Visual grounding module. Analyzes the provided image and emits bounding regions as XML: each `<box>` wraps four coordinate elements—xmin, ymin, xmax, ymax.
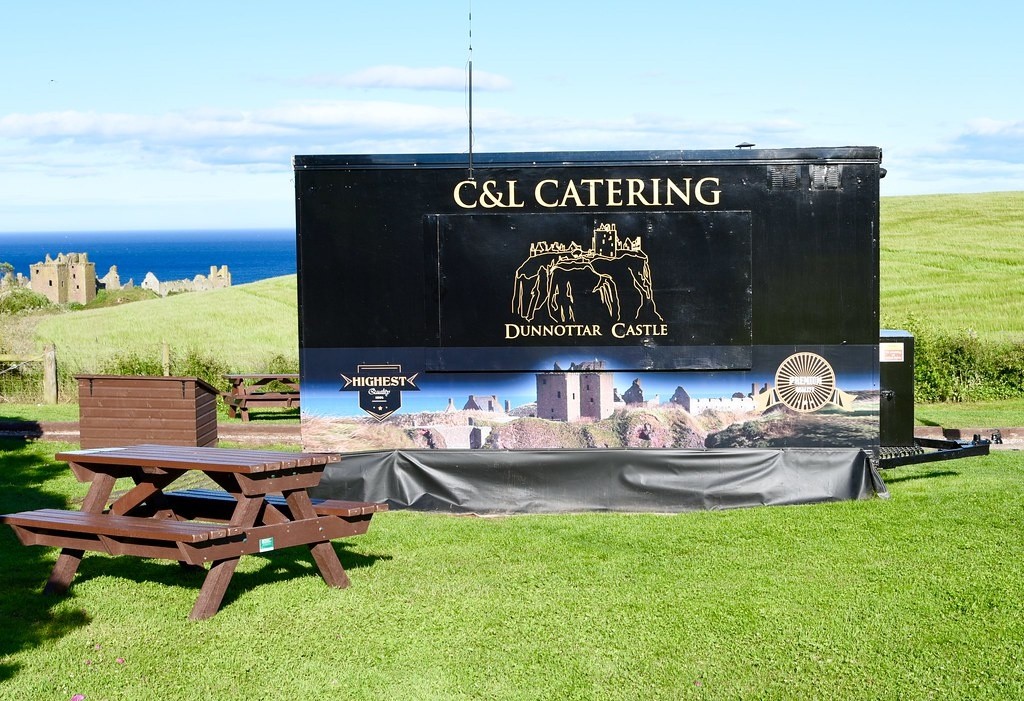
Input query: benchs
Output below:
<box><xmin>221</xmin><ymin>392</ymin><xmax>299</xmax><ymax>405</ymax></box>
<box><xmin>233</xmin><ymin>395</ymin><xmax>299</xmax><ymax>409</ymax></box>
<box><xmin>164</xmin><ymin>487</ymin><xmax>390</xmax><ymax>536</ymax></box>
<box><xmin>0</xmin><ymin>509</ymin><xmax>243</xmax><ymax>566</ymax></box>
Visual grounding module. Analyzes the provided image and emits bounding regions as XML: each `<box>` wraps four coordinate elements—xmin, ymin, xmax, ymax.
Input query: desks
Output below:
<box><xmin>222</xmin><ymin>374</ymin><xmax>299</xmax><ymax>422</ymax></box>
<box><xmin>42</xmin><ymin>445</ymin><xmax>350</xmax><ymax>622</ymax></box>
